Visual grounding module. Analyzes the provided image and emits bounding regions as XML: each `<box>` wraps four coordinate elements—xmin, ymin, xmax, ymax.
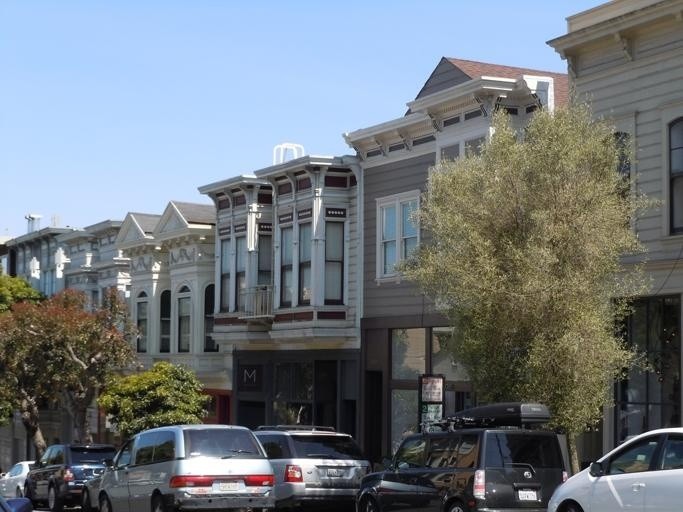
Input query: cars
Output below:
<box><xmin>0</xmin><ymin>461</ymin><xmax>35</xmax><ymax>498</ymax></box>
<box><xmin>81</xmin><ymin>476</ymin><xmax>103</xmax><ymax>512</ymax></box>
<box><xmin>620</xmin><ymin>388</ymin><xmax>652</xmax><ymax>433</ymax></box>
<box><xmin>548</xmin><ymin>427</ymin><xmax>683</xmax><ymax>512</ymax></box>
<box><xmin>0</xmin><ymin>495</ymin><xmax>33</xmax><ymax>512</ymax></box>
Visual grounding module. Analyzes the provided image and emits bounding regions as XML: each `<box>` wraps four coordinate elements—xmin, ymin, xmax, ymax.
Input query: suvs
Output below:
<box><xmin>355</xmin><ymin>402</ymin><xmax>568</xmax><ymax>512</ymax></box>
<box><xmin>253</xmin><ymin>425</ymin><xmax>373</xmax><ymax>512</ymax></box>
<box><xmin>24</xmin><ymin>444</ymin><xmax>118</xmax><ymax>512</ymax></box>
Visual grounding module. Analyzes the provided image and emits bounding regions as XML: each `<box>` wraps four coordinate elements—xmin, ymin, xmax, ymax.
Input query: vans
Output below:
<box><xmin>99</xmin><ymin>423</ymin><xmax>276</xmax><ymax>512</ymax></box>
